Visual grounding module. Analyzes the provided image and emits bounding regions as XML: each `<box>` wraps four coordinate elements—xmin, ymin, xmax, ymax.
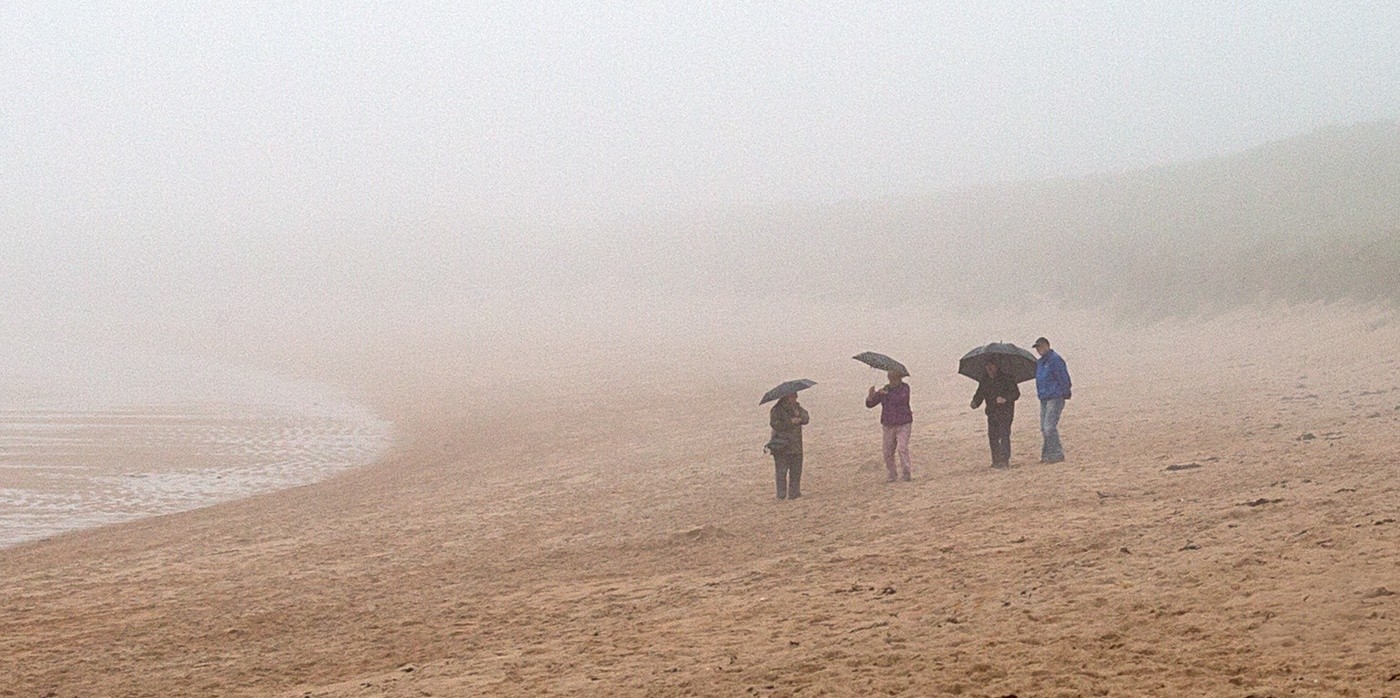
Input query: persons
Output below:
<box><xmin>865</xmin><ymin>370</ymin><xmax>914</xmax><ymax>483</ymax></box>
<box><xmin>1030</xmin><ymin>338</ymin><xmax>1073</xmax><ymax>463</ymax></box>
<box><xmin>967</xmin><ymin>355</ymin><xmax>1023</xmax><ymax>468</ymax></box>
<box><xmin>765</xmin><ymin>391</ymin><xmax>809</xmax><ymax>499</ymax></box>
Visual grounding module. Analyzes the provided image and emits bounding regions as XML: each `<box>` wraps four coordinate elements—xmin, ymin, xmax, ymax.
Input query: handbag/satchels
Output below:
<box><xmin>763</xmin><ymin>435</ymin><xmax>790</xmax><ymax>454</ymax></box>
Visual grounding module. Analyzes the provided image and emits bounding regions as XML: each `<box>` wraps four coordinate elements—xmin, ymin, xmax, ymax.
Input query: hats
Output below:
<box><xmin>1031</xmin><ymin>337</ymin><xmax>1048</xmax><ymax>348</ymax></box>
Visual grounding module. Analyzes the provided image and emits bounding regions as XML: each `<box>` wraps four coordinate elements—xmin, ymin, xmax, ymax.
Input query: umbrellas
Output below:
<box><xmin>852</xmin><ymin>351</ymin><xmax>911</xmax><ymax>391</ymax></box>
<box><xmin>758</xmin><ymin>377</ymin><xmax>818</xmax><ymax>418</ymax></box>
<box><xmin>958</xmin><ymin>339</ymin><xmax>1040</xmax><ymax>384</ymax></box>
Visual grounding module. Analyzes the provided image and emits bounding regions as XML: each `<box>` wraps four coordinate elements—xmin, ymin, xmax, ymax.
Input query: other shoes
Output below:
<box><xmin>1038</xmin><ymin>456</ymin><xmax>1064</xmax><ymax>465</ymax></box>
